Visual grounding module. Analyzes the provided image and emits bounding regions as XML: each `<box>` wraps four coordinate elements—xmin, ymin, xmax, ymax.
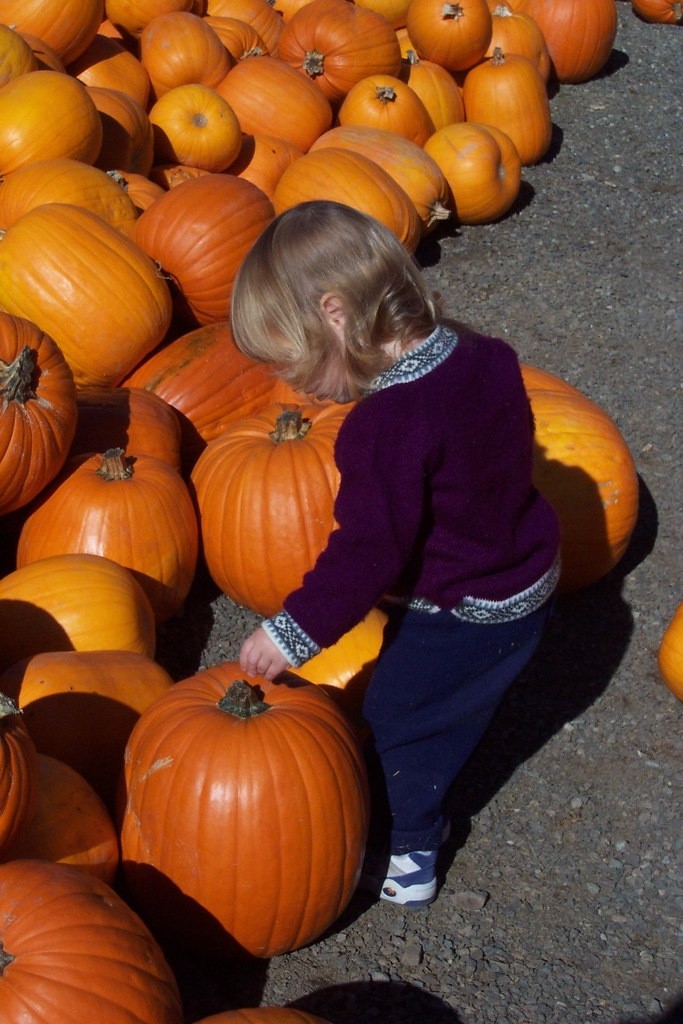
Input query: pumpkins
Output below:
<box><xmin>0</xmin><ymin>0</ymin><xmax>683</xmax><ymax>1024</ymax></box>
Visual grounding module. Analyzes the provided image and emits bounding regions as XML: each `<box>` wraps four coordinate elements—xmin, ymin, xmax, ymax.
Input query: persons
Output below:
<box><xmin>228</xmin><ymin>200</ymin><xmax>564</xmax><ymax>905</ymax></box>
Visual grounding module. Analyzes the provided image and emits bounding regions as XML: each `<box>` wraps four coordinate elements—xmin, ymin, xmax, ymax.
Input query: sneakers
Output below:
<box><xmin>360</xmin><ymin>849</ymin><xmax>441</xmax><ymax>907</ymax></box>
